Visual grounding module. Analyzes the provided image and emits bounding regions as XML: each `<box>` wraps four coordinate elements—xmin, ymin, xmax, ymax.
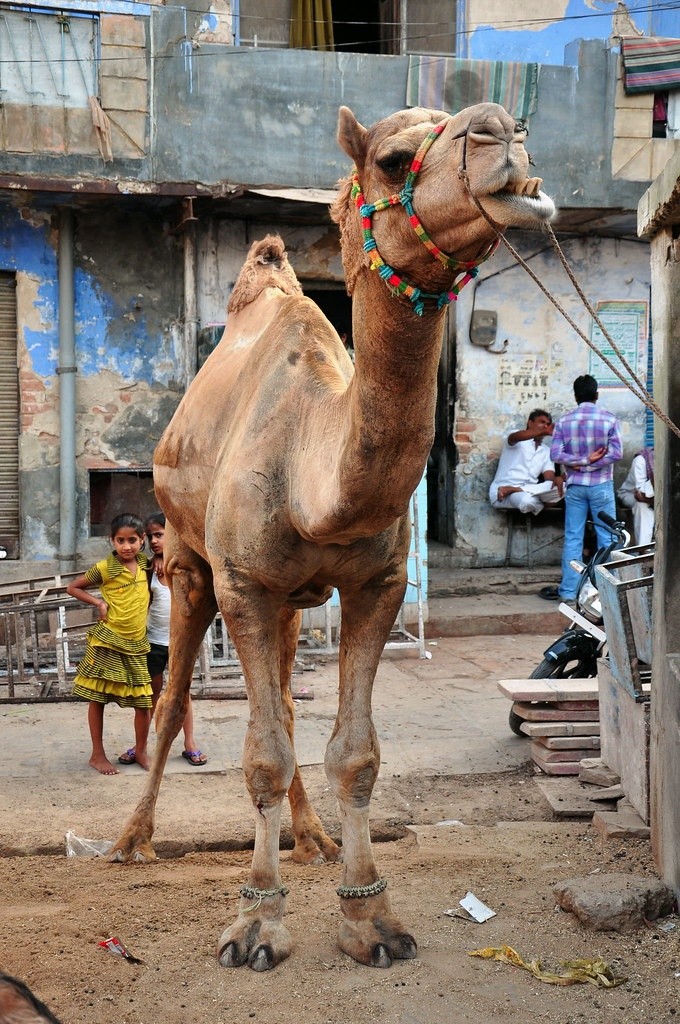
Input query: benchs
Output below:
<box><xmin>501</xmin><ymin>508</ymin><xmax>636</xmax><ymax>566</ymax></box>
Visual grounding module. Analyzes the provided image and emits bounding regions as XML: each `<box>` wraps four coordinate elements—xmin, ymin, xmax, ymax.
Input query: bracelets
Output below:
<box><xmin>96</xmin><ymin>599</ymin><xmax>103</xmax><ymax>608</ymax></box>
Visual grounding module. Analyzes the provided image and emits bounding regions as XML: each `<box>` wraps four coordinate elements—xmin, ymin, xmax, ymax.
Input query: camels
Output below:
<box><xmin>110</xmin><ymin>104</ymin><xmax>560</xmax><ymax>972</ymax></box>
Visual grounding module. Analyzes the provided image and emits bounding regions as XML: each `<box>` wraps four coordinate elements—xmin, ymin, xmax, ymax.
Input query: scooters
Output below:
<box><xmin>508</xmin><ymin>510</ymin><xmax>651</xmax><ymax>738</ymax></box>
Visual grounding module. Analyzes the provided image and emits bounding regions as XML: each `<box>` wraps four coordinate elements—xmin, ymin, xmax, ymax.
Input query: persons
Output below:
<box><xmin>488</xmin><ymin>408</ymin><xmax>567</xmax><ymax>515</ymax></box>
<box><xmin>118</xmin><ymin>512</ymin><xmax>208</xmax><ymax>765</ymax></box>
<box><xmin>340</xmin><ymin>332</ymin><xmax>355</xmax><ymax>364</ymax></box>
<box><xmin>66</xmin><ymin>513</ymin><xmax>164</xmax><ymax>775</ymax></box>
<box><xmin>616</xmin><ymin>446</ymin><xmax>656</xmax><ymax>555</ymax></box>
<box><xmin>549</xmin><ymin>374</ymin><xmax>627</xmax><ymax>606</ymax></box>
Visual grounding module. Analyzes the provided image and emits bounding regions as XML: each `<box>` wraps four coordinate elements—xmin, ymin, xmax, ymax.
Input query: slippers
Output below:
<box><xmin>539</xmin><ymin>585</ymin><xmax>560</xmax><ymax>600</ymax></box>
<box><xmin>118</xmin><ymin>748</ymin><xmax>136</xmax><ymax>764</ymax></box>
<box><xmin>181</xmin><ymin>750</ymin><xmax>208</xmax><ymax>765</ymax></box>
